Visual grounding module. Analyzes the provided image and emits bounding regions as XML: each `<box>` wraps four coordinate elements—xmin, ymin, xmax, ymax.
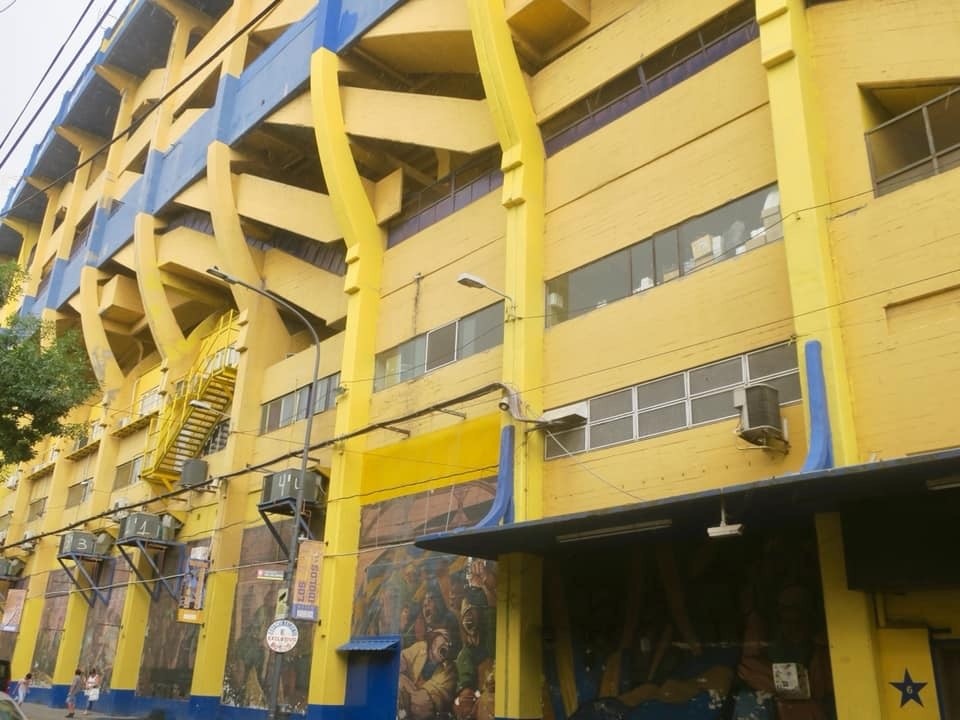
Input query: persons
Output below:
<box><xmin>82</xmin><ymin>668</ymin><xmax>101</xmax><ymax>714</ymax></box>
<box><xmin>64</xmin><ymin>670</ymin><xmax>82</xmax><ymax>719</ymax></box>
<box><xmin>11</xmin><ymin>673</ymin><xmax>33</xmax><ymax>706</ymax></box>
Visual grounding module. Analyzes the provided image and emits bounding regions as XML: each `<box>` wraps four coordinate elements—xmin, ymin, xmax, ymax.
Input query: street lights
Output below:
<box><xmin>206</xmin><ymin>266</ymin><xmax>320</xmax><ymax>717</ymax></box>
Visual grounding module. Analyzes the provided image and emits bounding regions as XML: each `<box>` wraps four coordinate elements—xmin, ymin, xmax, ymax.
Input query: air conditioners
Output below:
<box><xmin>113</xmin><ymin>497</ymin><xmax>128</xmax><ymax>522</ymax></box>
<box><xmin>7</xmin><ymin>476</ymin><xmax>17</xmax><ymax>489</ymax></box>
<box><xmin>119</xmin><ymin>417</ymin><xmax>129</xmax><ymax>426</ymax></box>
<box><xmin>191</xmin><ymin>545</ymin><xmax>210</xmax><ymax>561</ymax></box>
<box><xmin>22</xmin><ymin>531</ymin><xmax>34</xmax><ymax>550</ymax></box>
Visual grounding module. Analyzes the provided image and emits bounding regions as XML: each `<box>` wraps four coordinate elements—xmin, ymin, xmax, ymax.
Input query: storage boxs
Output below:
<box><xmin>593</xmin><ymin>190</ymin><xmax>787</xmax><ymax>308</ymax></box>
